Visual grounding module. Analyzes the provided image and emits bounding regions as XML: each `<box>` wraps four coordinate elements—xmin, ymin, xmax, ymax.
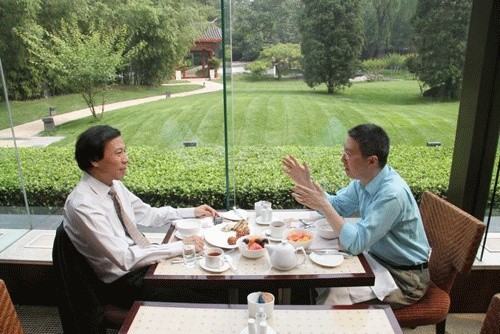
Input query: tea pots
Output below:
<box><xmin>264</xmin><ymin>240</ymin><xmax>306</xmax><ymax>271</ymax></box>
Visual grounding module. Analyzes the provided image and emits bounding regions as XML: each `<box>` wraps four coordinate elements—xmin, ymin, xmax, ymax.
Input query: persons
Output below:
<box><xmin>63</xmin><ymin>125</ymin><xmax>216</xmax><ymax>310</ymax></box>
<box><xmin>281</xmin><ymin>123</ymin><xmax>432</xmax><ymax>308</ymax></box>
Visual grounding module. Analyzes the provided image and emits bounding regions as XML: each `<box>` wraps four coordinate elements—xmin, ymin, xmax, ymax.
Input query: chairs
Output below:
<box><xmin>52</xmin><ymin>221</ymin><xmax>129</xmax><ymax>334</ymax></box>
<box><xmin>394</xmin><ymin>191</ymin><xmax>487</xmax><ymax>334</ymax></box>
<box><xmin>0</xmin><ymin>279</ymin><xmax>24</xmax><ymax>334</ymax></box>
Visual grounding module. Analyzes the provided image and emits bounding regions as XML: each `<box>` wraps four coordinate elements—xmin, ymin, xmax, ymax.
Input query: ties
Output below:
<box><xmin>108</xmin><ymin>187</ymin><xmax>150</xmax><ymax>246</ymax></box>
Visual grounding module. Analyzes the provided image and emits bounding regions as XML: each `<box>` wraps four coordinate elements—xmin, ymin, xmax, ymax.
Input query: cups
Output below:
<box><xmin>205</xmin><ymin>247</ymin><xmax>225</xmax><ymax>269</ymax></box>
<box><xmin>269</xmin><ymin>220</ymin><xmax>286</xmax><ymax>240</ymax></box>
<box><xmin>247</xmin><ymin>292</ymin><xmax>275</xmax><ymax>319</ymax></box>
<box><xmin>182</xmin><ymin>244</ymin><xmax>197</xmax><ymax>266</ymax></box>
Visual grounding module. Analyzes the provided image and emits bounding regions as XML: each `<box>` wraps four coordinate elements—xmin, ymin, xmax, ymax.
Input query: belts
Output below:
<box><xmin>391</xmin><ymin>261</ymin><xmax>430</xmax><ymax>271</ymax></box>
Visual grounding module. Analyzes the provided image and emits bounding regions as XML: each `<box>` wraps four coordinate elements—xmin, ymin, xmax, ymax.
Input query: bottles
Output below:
<box><xmin>261</xmin><ymin>204</ymin><xmax>272</xmax><ymax>222</ymax></box>
<box><xmin>248</xmin><ymin>317</ymin><xmax>267</xmax><ymax>334</ymax></box>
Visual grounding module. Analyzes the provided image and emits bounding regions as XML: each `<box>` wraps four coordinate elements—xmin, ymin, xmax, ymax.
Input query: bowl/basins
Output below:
<box><xmin>175</xmin><ymin>219</ymin><xmax>201</xmax><ymax>236</ymax></box>
<box><xmin>235</xmin><ymin>234</ymin><xmax>270</xmax><ymax>258</ymax></box>
<box><xmin>285</xmin><ymin>228</ymin><xmax>314</xmax><ymax>249</ymax></box>
<box><xmin>314</xmin><ymin>217</ymin><xmax>338</xmax><ymax>239</ymax></box>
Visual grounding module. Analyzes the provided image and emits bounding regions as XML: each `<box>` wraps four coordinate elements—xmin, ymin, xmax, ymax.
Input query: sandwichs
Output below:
<box><xmin>232</xmin><ymin>219</ymin><xmax>249</xmax><ymax>233</ymax></box>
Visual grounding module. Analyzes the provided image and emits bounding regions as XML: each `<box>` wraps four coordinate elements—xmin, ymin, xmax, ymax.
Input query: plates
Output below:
<box><xmin>204</xmin><ymin>221</ymin><xmax>255</xmax><ymax>249</ymax></box>
<box><xmin>309</xmin><ymin>251</ymin><xmax>344</xmax><ymax>267</ymax></box>
<box><xmin>256</xmin><ymin>216</ymin><xmax>276</xmax><ymax>225</ymax></box>
<box><xmin>174</xmin><ymin>229</ymin><xmax>204</xmax><ymax>240</ymax></box>
<box><xmin>239</xmin><ymin>324</ymin><xmax>277</xmax><ymax>334</ymax></box>
<box><xmin>199</xmin><ymin>257</ymin><xmax>231</xmax><ymax>272</ymax></box>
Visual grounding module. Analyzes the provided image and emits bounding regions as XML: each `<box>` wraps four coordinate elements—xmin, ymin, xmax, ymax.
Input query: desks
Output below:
<box><xmin>146</xmin><ymin>209</ymin><xmax>376</xmax><ymax>306</ymax></box>
<box><xmin>119</xmin><ymin>301</ymin><xmax>403</xmax><ymax>334</ymax></box>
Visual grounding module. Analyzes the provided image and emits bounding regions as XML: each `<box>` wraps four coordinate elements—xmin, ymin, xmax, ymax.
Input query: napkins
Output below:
<box><xmin>315</xmin><ymin>250</ymin><xmax>399</xmax><ymax>305</ymax></box>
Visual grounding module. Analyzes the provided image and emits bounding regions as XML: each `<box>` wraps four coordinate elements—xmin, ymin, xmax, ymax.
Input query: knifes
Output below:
<box><xmin>313</xmin><ymin>250</ymin><xmax>349</xmax><ymax>257</ymax></box>
